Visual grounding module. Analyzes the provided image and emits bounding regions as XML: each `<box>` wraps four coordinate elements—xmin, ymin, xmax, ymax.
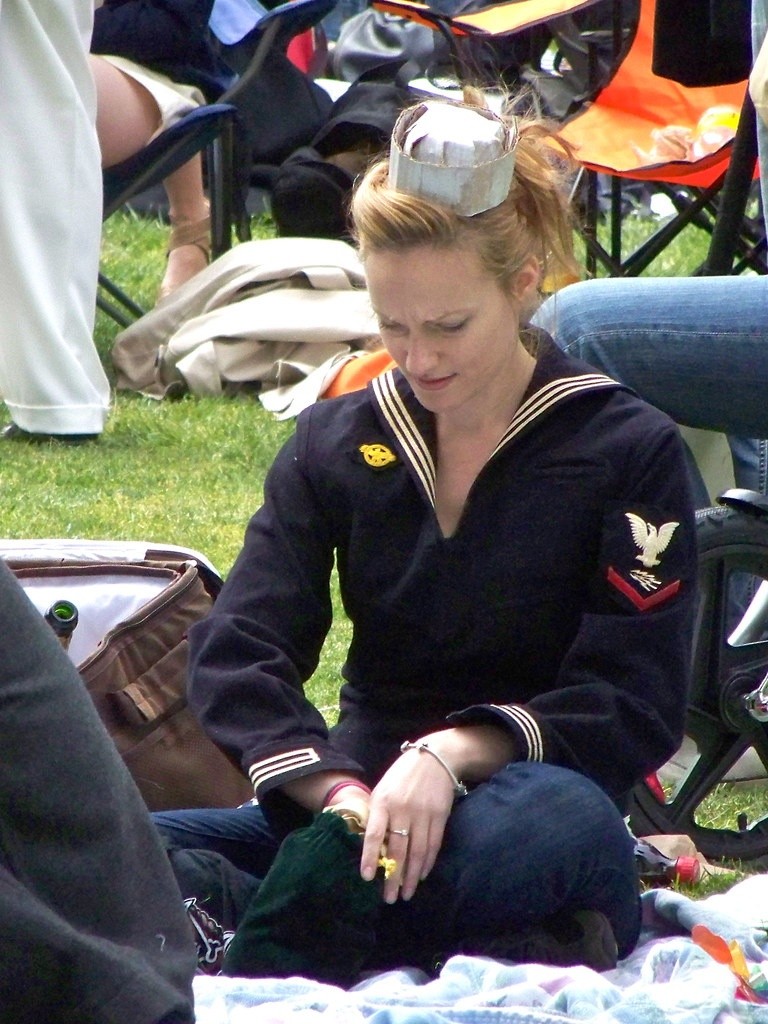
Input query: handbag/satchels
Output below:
<box><xmin>0</xmin><ymin>536</ymin><xmax>254</xmax><ymax>810</ymax></box>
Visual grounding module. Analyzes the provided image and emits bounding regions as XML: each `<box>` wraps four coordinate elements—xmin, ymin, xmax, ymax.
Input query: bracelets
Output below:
<box><xmin>323</xmin><ymin>780</ymin><xmax>372</xmax><ymax>811</ymax></box>
<box><xmin>400</xmin><ymin>740</ymin><xmax>468</xmax><ymax>796</ymax></box>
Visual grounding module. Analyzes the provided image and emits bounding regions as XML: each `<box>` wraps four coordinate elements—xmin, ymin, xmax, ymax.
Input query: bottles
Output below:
<box><xmin>45</xmin><ymin>600</ymin><xmax>78</xmax><ymax>650</ymax></box>
<box><xmin>632</xmin><ymin>836</ymin><xmax>697</xmax><ymax>889</ymax></box>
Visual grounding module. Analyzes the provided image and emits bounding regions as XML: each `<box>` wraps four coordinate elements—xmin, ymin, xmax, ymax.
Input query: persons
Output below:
<box><xmin>0</xmin><ymin>0</ymin><xmax>768</xmax><ymax>783</ymax></box>
<box><xmin>150</xmin><ymin>102</ymin><xmax>700</xmax><ymax>973</ymax></box>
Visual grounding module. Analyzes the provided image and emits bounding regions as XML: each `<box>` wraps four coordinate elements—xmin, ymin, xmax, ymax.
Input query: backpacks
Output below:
<box><xmin>279</xmin><ymin>58</ymin><xmax>420</xmax><ymax>255</ymax></box>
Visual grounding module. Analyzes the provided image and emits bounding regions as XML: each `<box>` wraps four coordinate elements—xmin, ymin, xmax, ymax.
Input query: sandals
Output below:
<box><xmin>155</xmin><ymin>210</ymin><xmax>212</xmax><ymax>308</ymax></box>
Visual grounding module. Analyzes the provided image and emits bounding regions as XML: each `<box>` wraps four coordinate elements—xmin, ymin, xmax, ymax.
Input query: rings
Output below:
<box><xmin>390</xmin><ymin>829</ymin><xmax>408</xmax><ymax>836</ymax></box>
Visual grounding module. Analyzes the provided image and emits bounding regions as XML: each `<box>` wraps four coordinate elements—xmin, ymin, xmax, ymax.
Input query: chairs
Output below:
<box><xmin>554</xmin><ymin>0</ymin><xmax>768</xmax><ymax>278</ymax></box>
<box><xmin>95</xmin><ymin>104</ymin><xmax>243</xmax><ymax>327</ymax></box>
